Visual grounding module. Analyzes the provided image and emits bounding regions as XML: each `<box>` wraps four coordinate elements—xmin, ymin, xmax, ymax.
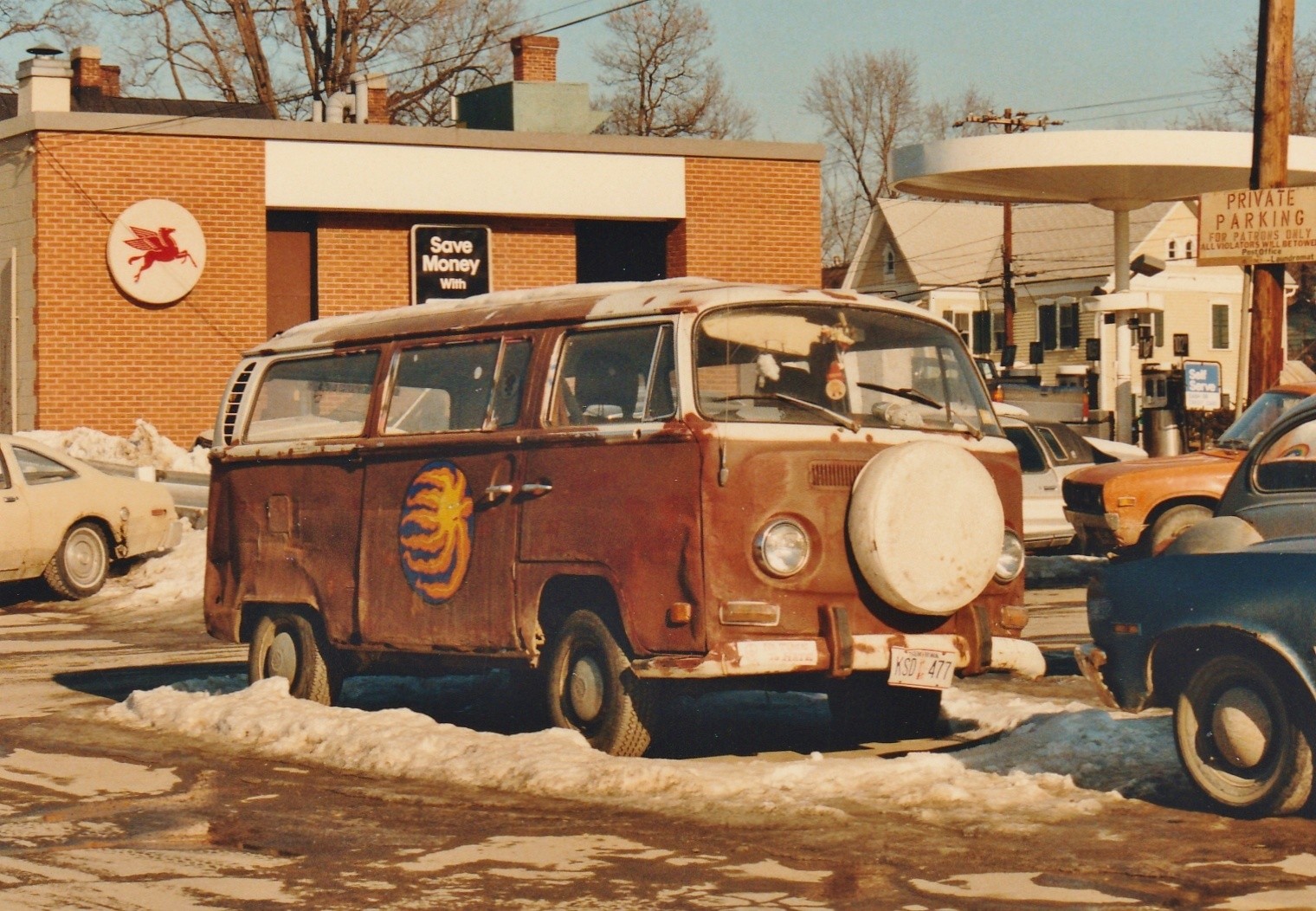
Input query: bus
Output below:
<box><xmin>201</xmin><ymin>276</ymin><xmax>1049</xmax><ymax>758</ymax></box>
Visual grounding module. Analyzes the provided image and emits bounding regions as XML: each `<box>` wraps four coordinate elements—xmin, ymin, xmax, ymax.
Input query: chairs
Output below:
<box><xmin>570</xmin><ymin>353</ymin><xmax>648</xmax><ymax>420</ymax></box>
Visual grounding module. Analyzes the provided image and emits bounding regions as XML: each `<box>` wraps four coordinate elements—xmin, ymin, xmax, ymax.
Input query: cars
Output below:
<box><xmin>1059</xmin><ymin>379</ymin><xmax>1316</xmax><ymax>558</ymax></box>
<box><xmin>1212</xmin><ymin>392</ymin><xmax>1316</xmax><ymax>540</ymax></box>
<box><xmin>0</xmin><ymin>433</ymin><xmax>183</xmax><ymax>602</ymax></box>
<box><xmin>1073</xmin><ymin>513</ymin><xmax>1315</xmax><ymax>820</ymax></box>
<box><xmin>913</xmin><ymin>397</ymin><xmax>1149</xmax><ymax>553</ymax></box>
